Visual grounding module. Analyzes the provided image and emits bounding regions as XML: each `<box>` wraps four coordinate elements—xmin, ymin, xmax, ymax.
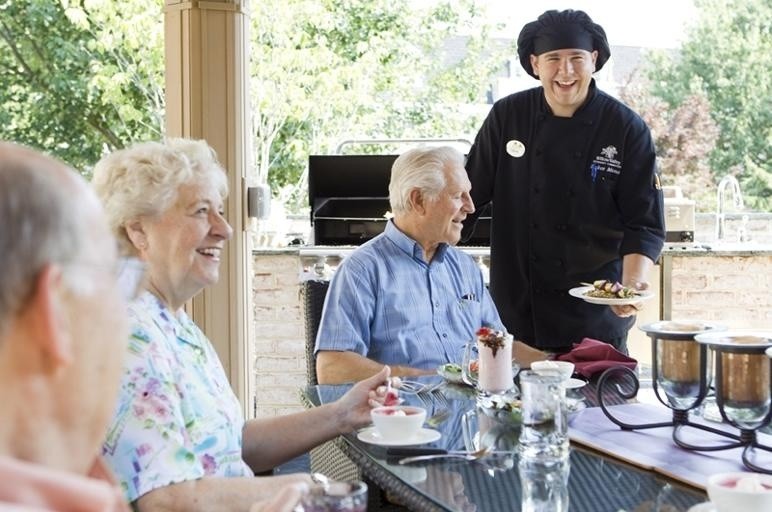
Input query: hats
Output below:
<box><xmin>517</xmin><ymin>8</ymin><xmax>611</xmax><ymax>80</ymax></box>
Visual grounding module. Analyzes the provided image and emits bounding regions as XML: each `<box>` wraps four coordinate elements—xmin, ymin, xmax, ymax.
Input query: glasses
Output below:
<box><xmin>107</xmin><ymin>255</ymin><xmax>146</xmax><ymax>299</ymax></box>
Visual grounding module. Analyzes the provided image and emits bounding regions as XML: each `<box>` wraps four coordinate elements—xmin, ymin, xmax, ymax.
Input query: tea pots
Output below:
<box><xmin>461</xmin><ymin>334</ymin><xmax>520</xmax><ymax>398</ymax></box>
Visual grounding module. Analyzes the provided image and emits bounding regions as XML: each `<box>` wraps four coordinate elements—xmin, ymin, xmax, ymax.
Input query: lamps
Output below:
<box><xmin>597</xmin><ymin>315</ymin><xmax>771</xmax><ymax>472</ymax></box>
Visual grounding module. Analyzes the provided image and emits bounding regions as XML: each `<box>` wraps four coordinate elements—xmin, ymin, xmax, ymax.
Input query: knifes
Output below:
<box><xmin>387</xmin><ymin>447</ymin><xmax>512</xmax><ymax>455</ymax></box>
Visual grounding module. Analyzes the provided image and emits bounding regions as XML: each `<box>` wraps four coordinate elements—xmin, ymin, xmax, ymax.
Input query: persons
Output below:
<box><xmin>0</xmin><ymin>139</ymin><xmax>131</xmax><ymax>510</ymax></box>
<box><xmin>313</xmin><ymin>145</ymin><xmax>552</xmax><ymax>386</ymax></box>
<box><xmin>87</xmin><ymin>137</ymin><xmax>402</xmax><ymax>511</ymax></box>
<box><xmin>458</xmin><ymin>8</ymin><xmax>665</xmax><ymax>370</ymax></box>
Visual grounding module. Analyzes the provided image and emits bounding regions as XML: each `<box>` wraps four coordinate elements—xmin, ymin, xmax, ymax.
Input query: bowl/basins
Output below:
<box><xmin>706</xmin><ymin>472</ymin><xmax>772</xmax><ymax>510</ymax></box>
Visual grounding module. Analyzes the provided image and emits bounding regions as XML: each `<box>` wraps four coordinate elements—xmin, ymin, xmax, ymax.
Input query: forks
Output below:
<box><xmin>399</xmin><ymin>449</ymin><xmax>487</xmax><ymax>465</ymax></box>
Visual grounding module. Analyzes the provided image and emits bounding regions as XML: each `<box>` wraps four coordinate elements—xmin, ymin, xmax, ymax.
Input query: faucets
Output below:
<box><xmin>717</xmin><ymin>175</ymin><xmax>743</xmax><ymax>241</ymax></box>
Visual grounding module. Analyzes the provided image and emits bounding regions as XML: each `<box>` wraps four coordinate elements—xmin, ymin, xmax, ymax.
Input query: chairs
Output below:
<box><xmin>299</xmin><ymin>277</ymin><xmax>362</xmax><ymax>482</ymax></box>
<box><xmin>716</xmin><ymin>174</ymin><xmax>768</xmax><ymax>244</ymax></box>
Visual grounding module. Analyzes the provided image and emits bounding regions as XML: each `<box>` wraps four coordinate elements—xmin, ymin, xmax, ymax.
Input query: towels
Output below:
<box><xmin>558</xmin><ymin>338</ymin><xmax>636</xmax><ymax>377</ymax></box>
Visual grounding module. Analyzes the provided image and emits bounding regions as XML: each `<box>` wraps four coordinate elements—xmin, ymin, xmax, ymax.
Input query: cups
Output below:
<box><xmin>519</xmin><ymin>371</ymin><xmax>570</xmax><ymax>461</ymax></box>
<box><xmin>461</xmin><ymin>392</ymin><xmax>520</xmax><ymax>465</ymax></box>
<box><xmin>370</xmin><ymin>405</ymin><xmax>426</xmax><ymax>439</ymax></box>
<box><xmin>531</xmin><ymin>360</ymin><xmax>575</xmax><ymax>383</ymax></box>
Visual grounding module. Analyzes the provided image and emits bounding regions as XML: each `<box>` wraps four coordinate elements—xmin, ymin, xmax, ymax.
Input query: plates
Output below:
<box><xmin>688</xmin><ymin>501</ymin><xmax>715</xmax><ymax>512</ymax></box>
<box><xmin>357</xmin><ymin>430</ymin><xmax>442</xmax><ymax>446</ymax></box>
<box><xmin>692</xmin><ymin>332</ymin><xmax>771</xmax><ymax>348</ymax></box>
<box><xmin>638</xmin><ymin>319</ymin><xmax>706</xmax><ymax>334</ymax></box>
<box><xmin>565</xmin><ymin>379</ymin><xmax>586</xmax><ymax>389</ymax></box>
<box><xmin>569</xmin><ymin>286</ymin><xmax>657</xmax><ymax>306</ymax></box>
